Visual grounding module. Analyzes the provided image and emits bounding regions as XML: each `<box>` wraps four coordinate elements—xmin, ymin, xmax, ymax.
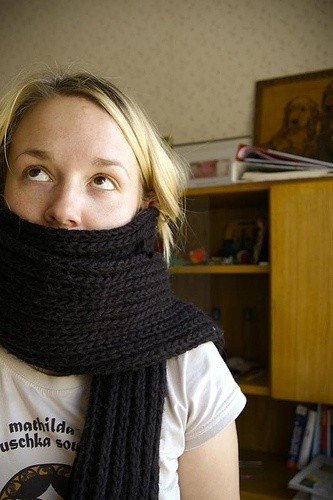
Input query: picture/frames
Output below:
<box><xmin>251</xmin><ymin>66</ymin><xmax>333</xmax><ymax>173</ymax></box>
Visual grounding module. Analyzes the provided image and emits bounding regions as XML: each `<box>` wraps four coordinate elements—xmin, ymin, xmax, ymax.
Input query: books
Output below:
<box><xmin>231</xmin><ymin>140</ymin><xmax>333</xmax><ymax>183</ymax></box>
<box><xmin>286</xmin><ymin>404</ymin><xmax>333</xmax><ymax>500</ymax></box>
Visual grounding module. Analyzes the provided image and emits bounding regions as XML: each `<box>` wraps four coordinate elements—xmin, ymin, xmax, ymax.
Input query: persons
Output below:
<box><xmin>1</xmin><ymin>73</ymin><xmax>248</xmax><ymax>499</ymax></box>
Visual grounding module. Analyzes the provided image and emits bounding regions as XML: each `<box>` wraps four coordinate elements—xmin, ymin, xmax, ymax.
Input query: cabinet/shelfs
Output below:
<box><xmin>170</xmin><ymin>174</ymin><xmax>333</xmax><ymax>500</ymax></box>
